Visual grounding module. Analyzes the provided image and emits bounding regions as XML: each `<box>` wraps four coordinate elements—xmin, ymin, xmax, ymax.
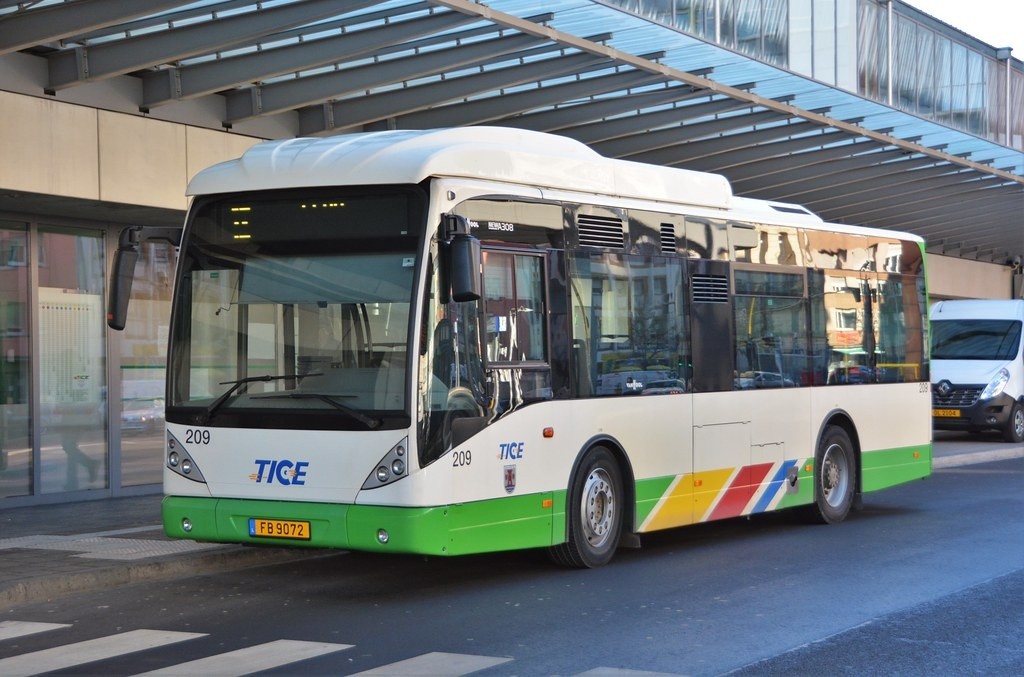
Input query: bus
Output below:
<box><xmin>107</xmin><ymin>124</ymin><xmax>935</xmax><ymax>571</ymax></box>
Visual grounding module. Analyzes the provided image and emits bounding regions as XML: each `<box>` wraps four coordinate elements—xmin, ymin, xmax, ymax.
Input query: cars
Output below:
<box><xmin>592</xmin><ymin>359</ymin><xmax>906</xmax><ymax>398</ymax></box>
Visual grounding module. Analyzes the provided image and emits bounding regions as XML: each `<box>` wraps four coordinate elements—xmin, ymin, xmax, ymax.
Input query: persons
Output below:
<box><xmin>55</xmin><ymin>357</ymin><xmax>100</xmax><ymax>493</ymax></box>
<box><xmin>433</xmin><ymin>319</ymin><xmax>486</xmax><ymax>399</ymax></box>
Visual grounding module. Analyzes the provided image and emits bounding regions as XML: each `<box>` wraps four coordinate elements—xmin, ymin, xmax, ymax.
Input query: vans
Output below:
<box><xmin>926</xmin><ymin>299</ymin><xmax>1024</xmax><ymax>445</ymax></box>
